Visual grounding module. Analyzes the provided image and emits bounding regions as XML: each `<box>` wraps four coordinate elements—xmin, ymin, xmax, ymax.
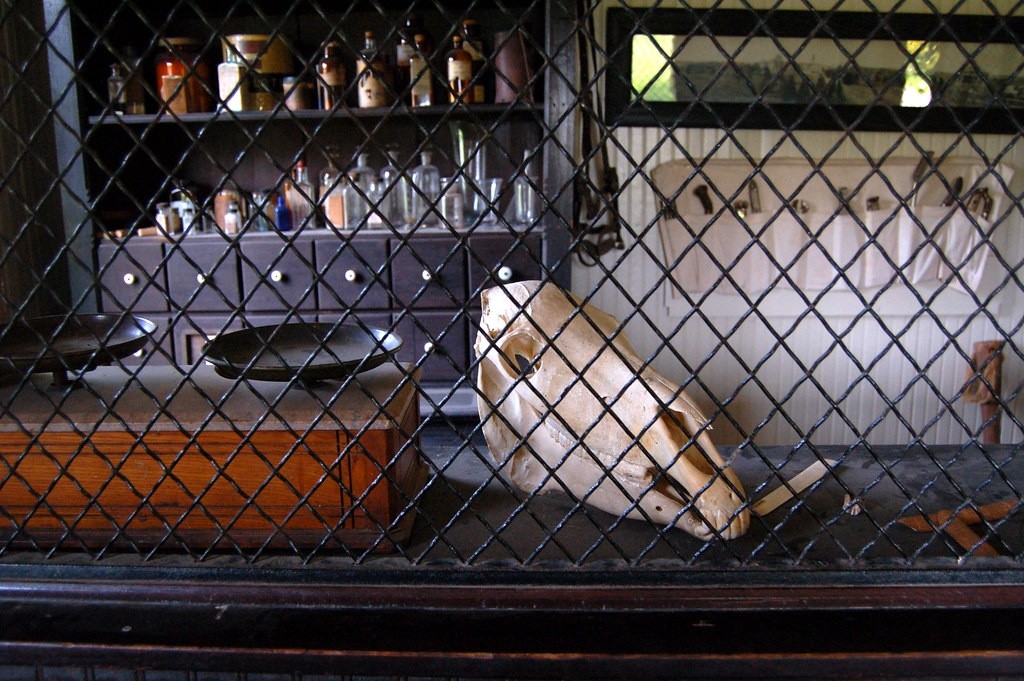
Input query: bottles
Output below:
<box><xmin>108</xmin><ymin>20</ymin><xmax>532</xmax><ymax>112</ymax></box>
<box><xmin>154</xmin><ymin>123</ymin><xmax>536</xmax><ymax>238</ymax></box>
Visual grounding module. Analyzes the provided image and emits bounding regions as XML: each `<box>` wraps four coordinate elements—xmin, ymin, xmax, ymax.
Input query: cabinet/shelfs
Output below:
<box><xmin>43</xmin><ymin>0</ymin><xmax>577</xmax><ymax>447</ymax></box>
